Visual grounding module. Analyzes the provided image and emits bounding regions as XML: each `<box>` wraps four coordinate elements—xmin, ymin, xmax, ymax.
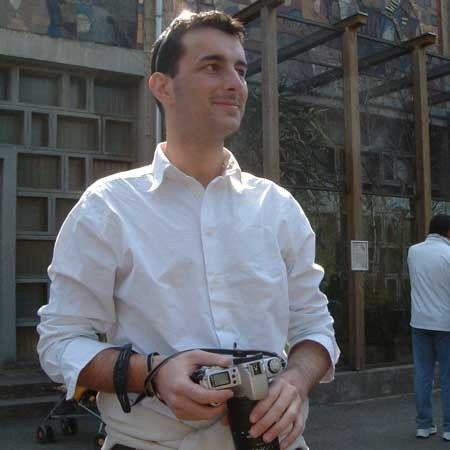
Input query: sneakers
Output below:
<box><xmin>417</xmin><ymin>425</ymin><xmax>450</xmax><ymax>440</ymax></box>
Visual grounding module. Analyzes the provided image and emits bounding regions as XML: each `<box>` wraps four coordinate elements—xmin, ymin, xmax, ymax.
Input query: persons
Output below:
<box><xmin>407</xmin><ymin>213</ymin><xmax>450</xmax><ymax>441</ymax></box>
<box><xmin>34</xmin><ymin>9</ymin><xmax>341</xmax><ymax>450</ymax></box>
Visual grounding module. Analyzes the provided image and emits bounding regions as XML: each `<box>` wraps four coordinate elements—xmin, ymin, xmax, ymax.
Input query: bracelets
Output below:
<box><xmin>148</xmin><ymin>351</ymin><xmax>159</xmax><ymax>402</ymax></box>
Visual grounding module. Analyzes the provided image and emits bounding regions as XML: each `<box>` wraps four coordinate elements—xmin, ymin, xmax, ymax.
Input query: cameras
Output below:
<box><xmin>190</xmin><ymin>353</ymin><xmax>281</xmax><ymax>450</ymax></box>
